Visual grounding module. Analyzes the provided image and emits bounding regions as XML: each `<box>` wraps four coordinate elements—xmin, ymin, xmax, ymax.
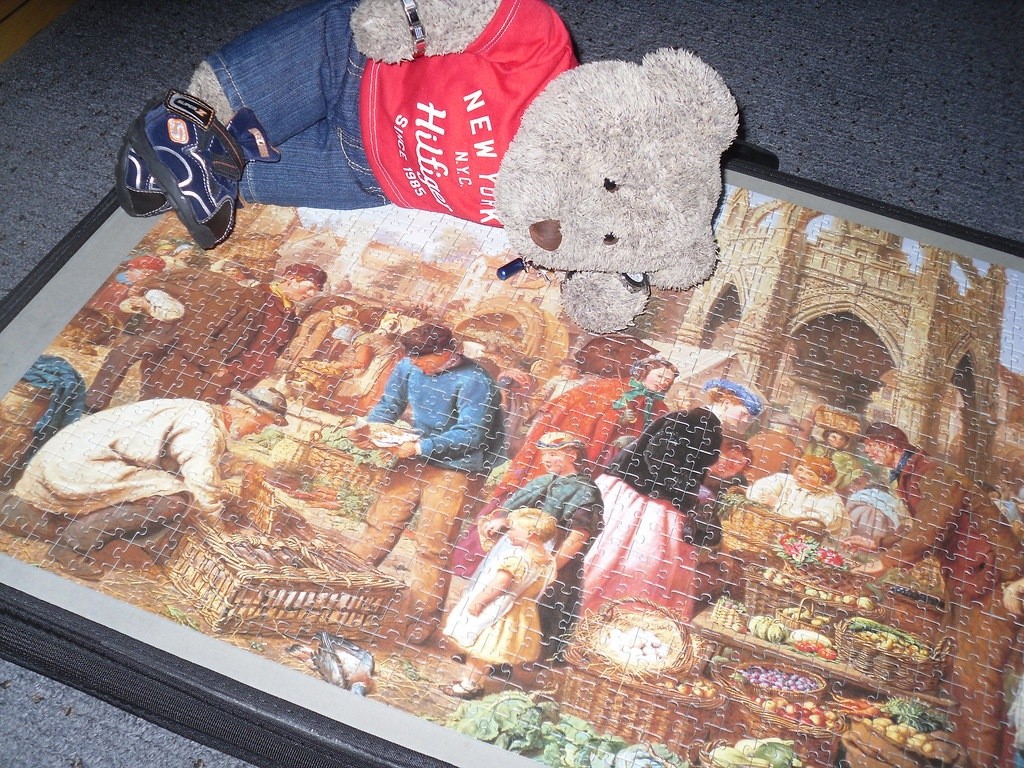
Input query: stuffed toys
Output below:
<box><xmin>114</xmin><ymin>0</ymin><xmax>739</xmax><ymax>334</ymax></box>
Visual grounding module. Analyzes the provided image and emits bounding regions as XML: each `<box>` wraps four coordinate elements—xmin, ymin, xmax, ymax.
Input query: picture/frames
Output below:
<box><xmin>0</xmin><ymin>158</ymin><xmax>1024</xmax><ymax>768</ymax></box>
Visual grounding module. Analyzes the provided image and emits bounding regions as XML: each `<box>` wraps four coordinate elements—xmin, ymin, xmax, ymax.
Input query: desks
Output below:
<box><xmin>690</xmin><ymin>605</ymin><xmax>957</xmax><ymax>708</ymax></box>
